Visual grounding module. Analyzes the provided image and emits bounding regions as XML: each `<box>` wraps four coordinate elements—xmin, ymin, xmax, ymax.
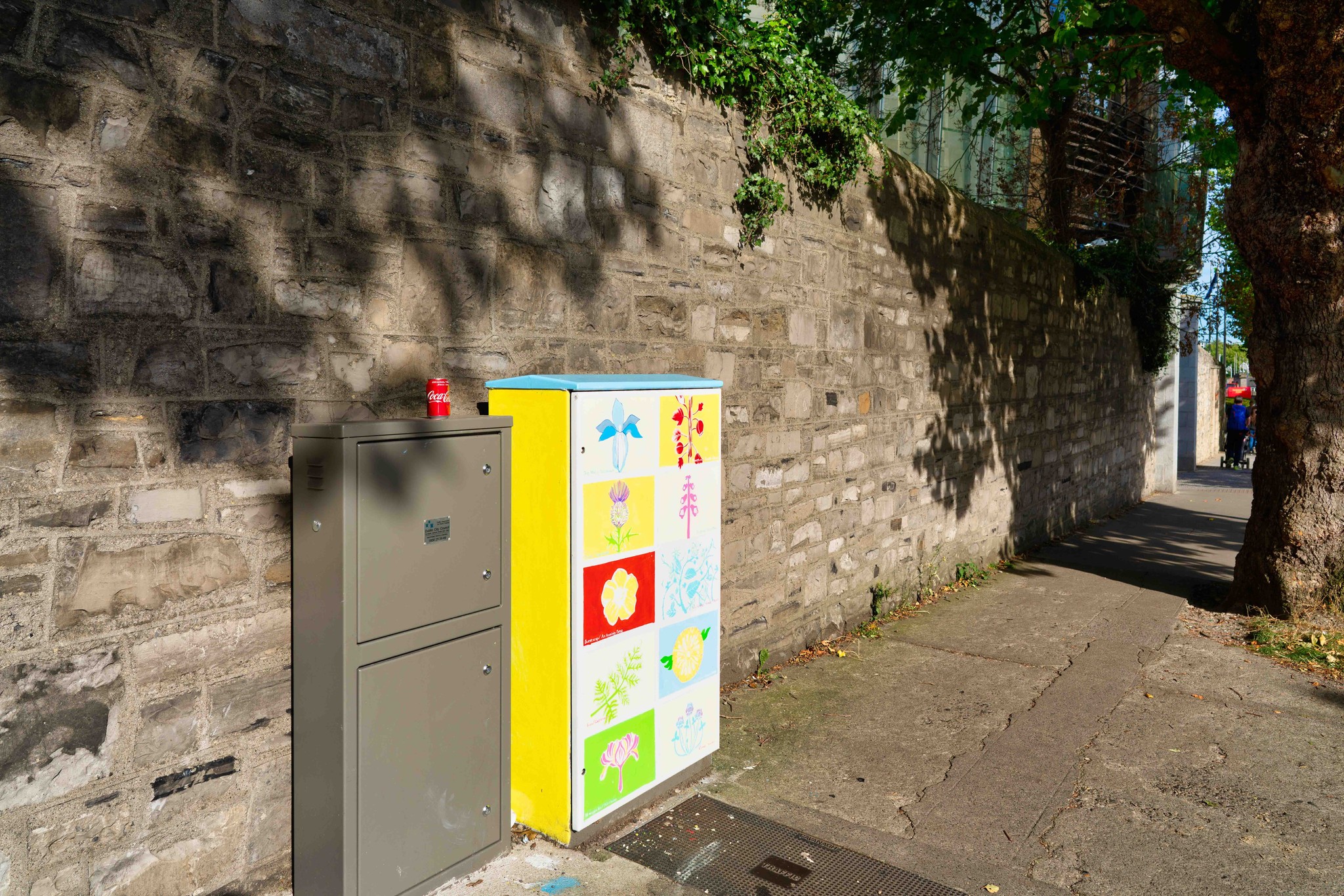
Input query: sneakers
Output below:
<box><xmin>1226</xmin><ymin>457</ymin><xmax>1234</xmax><ymax>468</ymax></box>
<box><xmin>1234</xmin><ymin>466</ymin><xmax>1243</xmax><ymax>470</ymax></box>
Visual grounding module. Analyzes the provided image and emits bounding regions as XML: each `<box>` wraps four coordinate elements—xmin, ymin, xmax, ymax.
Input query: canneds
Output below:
<box><xmin>426</xmin><ymin>378</ymin><xmax>451</xmax><ymax>418</ymax></box>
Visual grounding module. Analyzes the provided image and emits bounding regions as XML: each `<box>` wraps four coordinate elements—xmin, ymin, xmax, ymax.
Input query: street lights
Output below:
<box><xmin>1239</xmin><ymin>362</ymin><xmax>1248</xmax><ymax>387</ymax></box>
<box><xmin>1236</xmin><ymin>341</ymin><xmax>1248</xmax><ymax>364</ymax></box>
<box><xmin>1226</xmin><ymin>366</ymin><xmax>1232</xmax><ymax>378</ymax></box>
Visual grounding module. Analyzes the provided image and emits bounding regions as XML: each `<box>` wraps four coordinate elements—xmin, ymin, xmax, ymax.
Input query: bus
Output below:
<box><xmin>1226</xmin><ymin>383</ymin><xmax>1238</xmax><ymax>397</ymax></box>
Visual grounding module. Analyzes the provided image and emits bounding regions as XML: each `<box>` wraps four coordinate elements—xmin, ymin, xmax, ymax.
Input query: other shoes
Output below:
<box><xmin>1249</xmin><ymin>452</ymin><xmax>1253</xmax><ymax>455</ymax></box>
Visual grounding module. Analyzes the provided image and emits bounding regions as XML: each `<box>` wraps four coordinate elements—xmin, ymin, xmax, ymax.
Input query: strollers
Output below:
<box><xmin>1220</xmin><ymin>425</ymin><xmax>1249</xmax><ymax>469</ymax></box>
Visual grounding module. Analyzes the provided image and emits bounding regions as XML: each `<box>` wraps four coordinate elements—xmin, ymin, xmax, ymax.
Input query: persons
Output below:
<box><xmin>1225</xmin><ymin>396</ymin><xmax>1251</xmax><ymax>471</ymax></box>
<box><xmin>1246</xmin><ymin>402</ymin><xmax>1258</xmax><ymax>455</ymax></box>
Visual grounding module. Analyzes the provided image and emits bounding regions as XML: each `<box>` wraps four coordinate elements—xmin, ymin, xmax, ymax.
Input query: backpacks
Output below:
<box><xmin>1228</xmin><ymin>404</ymin><xmax>1249</xmax><ymax>436</ymax></box>
